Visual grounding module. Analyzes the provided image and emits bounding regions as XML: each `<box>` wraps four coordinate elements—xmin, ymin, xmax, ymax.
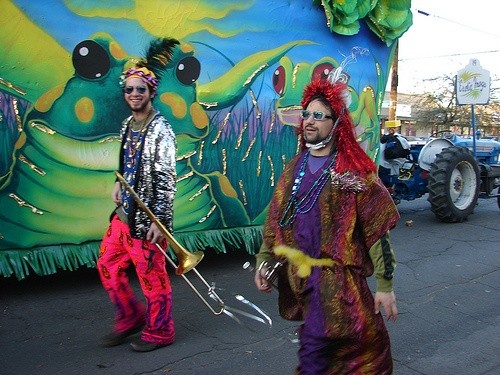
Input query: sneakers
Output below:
<box><xmin>97</xmin><ymin>323</ymin><xmax>160</xmax><ymax>352</ymax></box>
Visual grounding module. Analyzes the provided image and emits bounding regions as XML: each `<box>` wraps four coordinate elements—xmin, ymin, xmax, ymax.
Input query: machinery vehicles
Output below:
<box><xmin>377</xmin><ymin>103</ymin><xmax>500</xmax><ymax>224</ymax></box>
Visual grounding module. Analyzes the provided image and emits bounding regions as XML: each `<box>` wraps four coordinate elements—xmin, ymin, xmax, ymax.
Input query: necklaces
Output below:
<box><xmin>279</xmin><ymin>150</ymin><xmax>340</xmax><ymax>228</ymax></box>
<box><xmin>129</xmin><ymin>109</ymin><xmax>151</xmax><ymax>158</ymax></box>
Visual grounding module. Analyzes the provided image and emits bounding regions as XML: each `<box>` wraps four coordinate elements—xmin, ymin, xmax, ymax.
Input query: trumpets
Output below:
<box><xmin>257</xmin><ymin>259</ymin><xmax>283</xmax><ymax>292</ymax></box>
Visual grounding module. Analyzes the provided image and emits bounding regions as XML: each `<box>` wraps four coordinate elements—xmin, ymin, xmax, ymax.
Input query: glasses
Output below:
<box><xmin>299</xmin><ymin>110</ymin><xmax>333</xmax><ymax>121</ymax></box>
<box><xmin>122</xmin><ymin>85</ymin><xmax>147</xmax><ymax>95</ymax></box>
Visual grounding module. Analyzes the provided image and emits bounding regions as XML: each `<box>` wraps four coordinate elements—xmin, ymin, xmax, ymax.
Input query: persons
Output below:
<box><xmin>98</xmin><ymin>67</ymin><xmax>177</xmax><ymax>353</ymax></box>
<box><xmin>255</xmin><ymin>93</ymin><xmax>398</xmax><ymax>375</ymax></box>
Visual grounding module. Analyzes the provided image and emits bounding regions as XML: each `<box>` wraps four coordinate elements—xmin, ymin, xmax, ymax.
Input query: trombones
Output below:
<box><xmin>114</xmin><ymin>170</ymin><xmax>224</xmax><ymax>317</ymax></box>
